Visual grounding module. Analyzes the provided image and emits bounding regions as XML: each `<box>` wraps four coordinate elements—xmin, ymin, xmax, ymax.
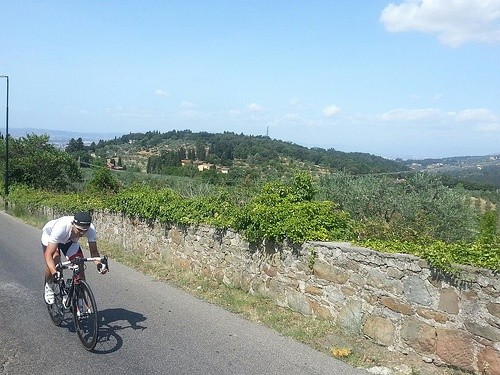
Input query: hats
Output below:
<box><xmin>74</xmin><ymin>212</ymin><xmax>91</xmax><ymax>229</ymax></box>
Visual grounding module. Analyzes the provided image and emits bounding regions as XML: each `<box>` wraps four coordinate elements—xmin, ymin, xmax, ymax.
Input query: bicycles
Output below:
<box><xmin>43</xmin><ymin>255</ymin><xmax>109</xmax><ymax>352</ymax></box>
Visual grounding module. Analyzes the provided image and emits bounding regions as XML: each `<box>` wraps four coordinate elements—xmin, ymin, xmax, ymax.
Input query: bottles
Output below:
<box><xmin>65</xmin><ymin>279</ymin><xmax>72</xmax><ymax>294</ymax></box>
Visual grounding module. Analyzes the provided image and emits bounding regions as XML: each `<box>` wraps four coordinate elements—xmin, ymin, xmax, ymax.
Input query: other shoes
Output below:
<box><xmin>76</xmin><ymin>297</ymin><xmax>86</xmax><ymax>317</ymax></box>
<box><xmin>44</xmin><ymin>282</ymin><xmax>56</xmax><ymax>305</ymax></box>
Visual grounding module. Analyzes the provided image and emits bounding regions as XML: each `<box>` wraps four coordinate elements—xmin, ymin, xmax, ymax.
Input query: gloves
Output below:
<box><xmin>52</xmin><ymin>273</ymin><xmax>64</xmax><ymax>284</ymax></box>
<box><xmin>97</xmin><ymin>263</ymin><xmax>108</xmax><ymax>274</ymax></box>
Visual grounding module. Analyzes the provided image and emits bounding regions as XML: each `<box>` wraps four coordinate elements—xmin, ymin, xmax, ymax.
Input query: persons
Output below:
<box><xmin>40</xmin><ymin>212</ymin><xmax>108</xmax><ymax>318</ymax></box>
